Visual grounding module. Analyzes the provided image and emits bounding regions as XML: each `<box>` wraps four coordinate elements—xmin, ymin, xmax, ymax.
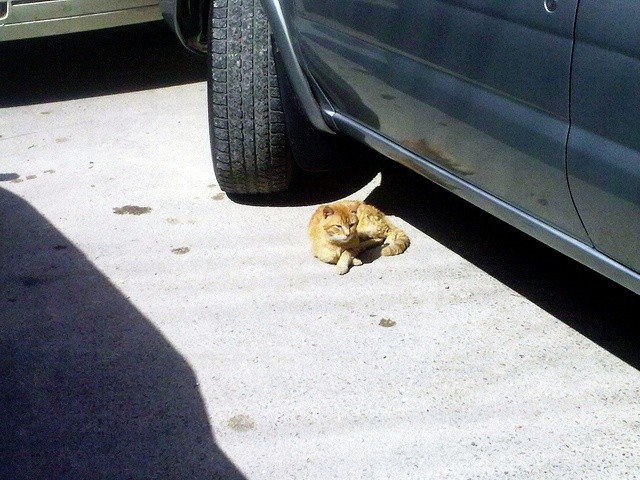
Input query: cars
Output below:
<box><xmin>0</xmin><ymin>0</ymin><xmax>212</xmax><ymax>71</ymax></box>
<box><xmin>158</xmin><ymin>0</ymin><xmax>640</xmax><ymax>294</ymax></box>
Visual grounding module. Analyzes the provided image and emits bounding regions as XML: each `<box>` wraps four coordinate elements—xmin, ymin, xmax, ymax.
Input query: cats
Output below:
<box><xmin>307</xmin><ymin>199</ymin><xmax>410</xmax><ymax>275</ymax></box>
